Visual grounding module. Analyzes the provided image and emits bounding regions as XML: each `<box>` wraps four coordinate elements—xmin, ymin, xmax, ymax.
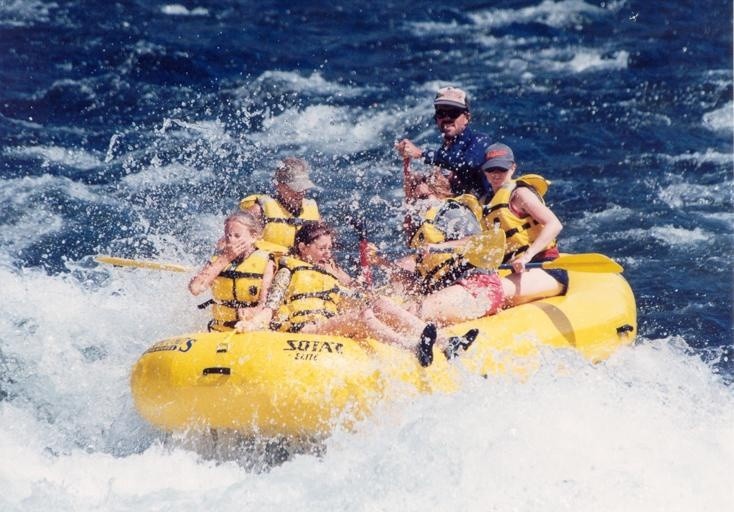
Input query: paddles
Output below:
<box><xmin>499</xmin><ymin>253</ymin><xmax>625</xmax><ymax>274</ymax></box>
<box><xmin>94</xmin><ymin>257</ymin><xmax>208</xmax><ymax>274</ymax></box>
<box><xmin>333</xmin><ymin>227</ymin><xmax>506</xmax><ymax>270</ymax></box>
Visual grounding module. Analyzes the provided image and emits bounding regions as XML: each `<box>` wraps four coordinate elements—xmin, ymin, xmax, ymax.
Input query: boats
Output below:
<box><xmin>127</xmin><ymin>250</ymin><xmax>637</xmax><ymax>435</ymax></box>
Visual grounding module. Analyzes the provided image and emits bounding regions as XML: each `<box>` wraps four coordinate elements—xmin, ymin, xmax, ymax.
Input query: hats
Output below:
<box><xmin>277</xmin><ymin>160</ymin><xmax>315</xmax><ymax>192</ymax></box>
<box><xmin>480</xmin><ymin>142</ymin><xmax>514</xmax><ymax>170</ymax></box>
<box><xmin>434</xmin><ymin>87</ymin><xmax>468</xmax><ymax>108</ymax></box>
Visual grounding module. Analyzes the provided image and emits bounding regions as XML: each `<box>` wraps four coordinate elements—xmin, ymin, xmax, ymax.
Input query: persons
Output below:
<box><xmin>391</xmin><ymin>86</ymin><xmax>494</xmax><ymax>204</ymax></box>
<box><xmin>239</xmin><ymin>158</ymin><xmax>323</xmax><ymax>262</ymax></box>
<box><xmin>187</xmin><ymin>208</ymin><xmax>278</xmax><ymax>335</ymax></box>
<box><xmin>363</xmin><ymin>168</ymin><xmax>503</xmax><ymax>328</ymax></box>
<box><xmin>235</xmin><ymin>224</ymin><xmax>481</xmax><ymax>368</ymax></box>
<box><xmin>470</xmin><ymin>139</ymin><xmax>568</xmax><ymax>310</ymax></box>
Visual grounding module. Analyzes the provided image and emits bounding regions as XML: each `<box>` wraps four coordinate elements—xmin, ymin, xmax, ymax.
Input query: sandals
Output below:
<box><xmin>416</xmin><ymin>323</ymin><xmax>436</xmax><ymax>367</ymax></box>
<box><xmin>442</xmin><ymin>328</ymin><xmax>480</xmax><ymax>362</ymax></box>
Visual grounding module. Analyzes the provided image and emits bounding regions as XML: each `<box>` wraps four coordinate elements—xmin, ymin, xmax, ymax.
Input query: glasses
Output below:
<box><xmin>436</xmin><ymin>109</ymin><xmax>460</xmax><ymax>119</ymax></box>
<box><xmin>486</xmin><ymin>166</ymin><xmax>508</xmax><ymax>172</ymax></box>
